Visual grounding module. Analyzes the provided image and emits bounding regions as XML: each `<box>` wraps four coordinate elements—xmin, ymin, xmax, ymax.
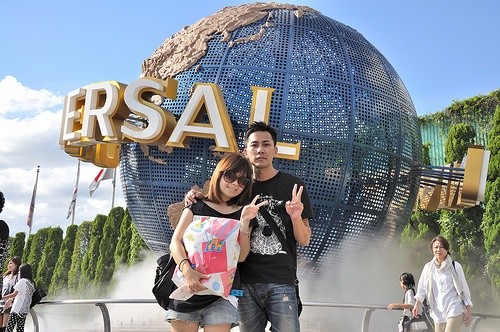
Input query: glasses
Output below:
<box><xmin>224</xmin><ymin>170</ymin><xmax>250</xmax><ymax>188</ymax></box>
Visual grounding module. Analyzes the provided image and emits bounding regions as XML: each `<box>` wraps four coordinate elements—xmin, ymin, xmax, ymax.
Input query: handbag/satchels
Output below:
<box><xmin>398</xmin><ymin>316</ymin><xmax>436</xmax><ymax>332</ymax></box>
<box><xmin>296</xmin><ymin>296</ymin><xmax>302</xmax><ymax>317</ymax></box>
<box><xmin>152</xmin><ymin>254</ymin><xmax>220</xmax><ymax>312</ymax></box>
